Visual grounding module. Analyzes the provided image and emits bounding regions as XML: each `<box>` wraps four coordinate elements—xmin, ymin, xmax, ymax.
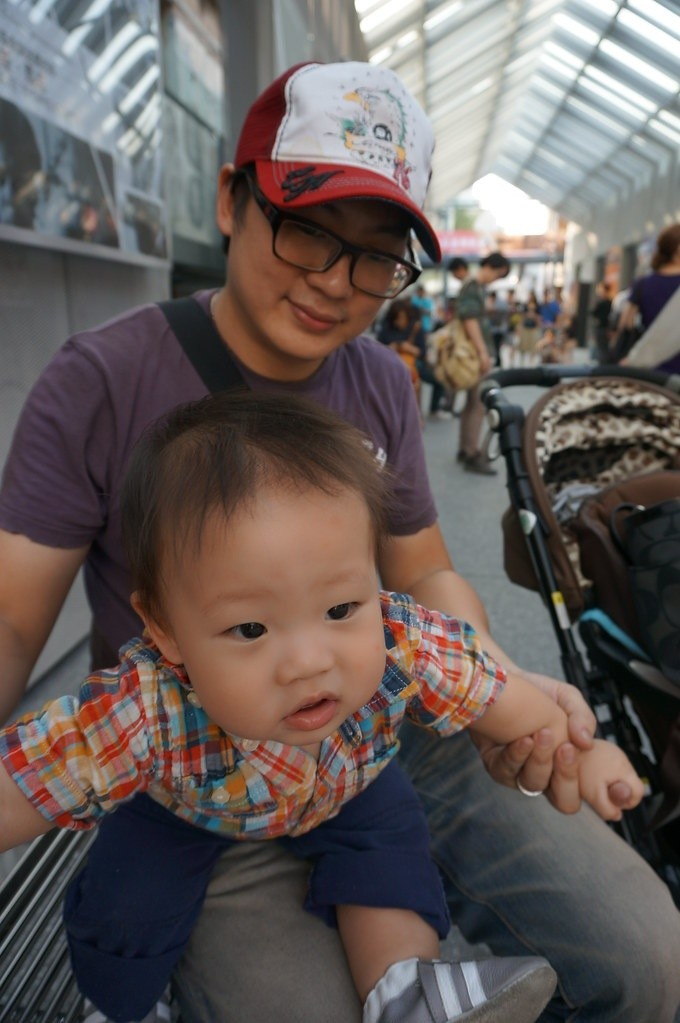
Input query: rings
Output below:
<box><xmin>515</xmin><ymin>776</ymin><xmax>544</xmax><ymax>797</ymax></box>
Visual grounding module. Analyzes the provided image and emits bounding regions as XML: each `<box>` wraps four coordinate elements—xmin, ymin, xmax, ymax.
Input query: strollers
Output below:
<box><xmin>480</xmin><ymin>361</ymin><xmax>680</xmax><ymax>902</ymax></box>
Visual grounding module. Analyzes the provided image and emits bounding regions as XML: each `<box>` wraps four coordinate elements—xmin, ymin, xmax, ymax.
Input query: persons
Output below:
<box><xmin>0</xmin><ymin>394</ymin><xmax>646</xmax><ymax>1023</ymax></box>
<box><xmin>375</xmin><ymin>220</ymin><xmax>680</xmax><ymax>476</ymax></box>
<box><xmin>0</xmin><ymin>62</ymin><xmax>680</xmax><ymax>1023</ymax></box>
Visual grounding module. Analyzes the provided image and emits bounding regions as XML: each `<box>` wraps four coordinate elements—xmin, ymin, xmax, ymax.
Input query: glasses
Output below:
<box><xmin>239</xmin><ymin>168</ymin><xmax>422</xmax><ymax>299</ymax></box>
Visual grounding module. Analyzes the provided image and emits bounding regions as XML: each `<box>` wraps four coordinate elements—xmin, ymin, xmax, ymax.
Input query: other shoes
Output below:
<box><xmin>457</xmin><ymin>449</ymin><xmax>467</xmax><ymax>463</ymax></box>
<box><xmin>378</xmin><ymin>955</ymin><xmax>558</xmax><ymax>1023</ymax></box>
<box><xmin>465</xmin><ymin>457</ymin><xmax>498</xmax><ymax>475</ymax></box>
<box><xmin>84</xmin><ymin>984</ymin><xmax>172</xmax><ymax>1023</ymax></box>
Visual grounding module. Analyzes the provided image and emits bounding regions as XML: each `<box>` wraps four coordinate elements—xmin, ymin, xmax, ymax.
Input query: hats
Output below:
<box><xmin>234</xmin><ymin>60</ymin><xmax>442</xmax><ymax>263</ymax></box>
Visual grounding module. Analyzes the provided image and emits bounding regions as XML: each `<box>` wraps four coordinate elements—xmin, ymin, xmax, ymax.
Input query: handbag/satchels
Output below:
<box><xmin>434</xmin><ymin>327</ymin><xmax>479</xmax><ymax>393</ymax></box>
<box><xmin>609</xmin><ymin>500</ymin><xmax>680</xmax><ymax>685</ymax></box>
